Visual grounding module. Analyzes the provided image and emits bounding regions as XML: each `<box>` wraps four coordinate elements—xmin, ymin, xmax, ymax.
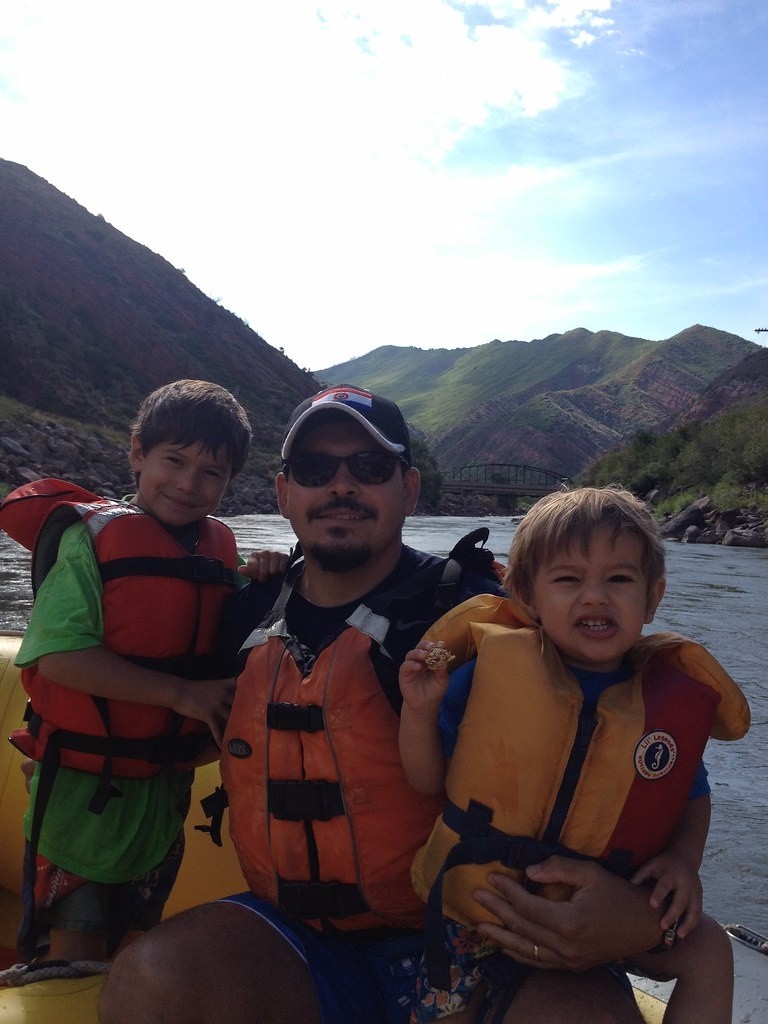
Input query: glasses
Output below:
<box><xmin>289</xmin><ymin>452</ymin><xmax>409</xmax><ymax>486</ymax></box>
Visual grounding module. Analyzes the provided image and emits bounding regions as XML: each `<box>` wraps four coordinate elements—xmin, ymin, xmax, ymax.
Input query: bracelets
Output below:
<box><xmin>646</xmin><ymin>881</ymin><xmax>680</xmax><ymax>955</ymax></box>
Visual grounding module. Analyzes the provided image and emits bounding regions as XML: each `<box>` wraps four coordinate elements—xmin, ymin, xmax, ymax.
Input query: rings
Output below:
<box><xmin>534</xmin><ymin>945</ymin><xmax>539</xmax><ymax>960</ymax></box>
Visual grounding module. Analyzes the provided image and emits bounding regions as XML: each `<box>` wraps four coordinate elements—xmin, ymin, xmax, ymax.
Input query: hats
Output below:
<box><xmin>281</xmin><ymin>383</ymin><xmax>410</xmax><ymax>466</ymax></box>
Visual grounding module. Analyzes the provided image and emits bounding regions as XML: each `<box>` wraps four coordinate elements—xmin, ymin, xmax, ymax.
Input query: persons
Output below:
<box><xmin>399</xmin><ymin>491</ymin><xmax>751</xmax><ymax>1024</ymax></box>
<box><xmin>0</xmin><ymin>379</ymin><xmax>289</xmax><ymax>963</ymax></box>
<box><xmin>20</xmin><ymin>386</ymin><xmax>680</xmax><ymax>1024</ymax></box>
